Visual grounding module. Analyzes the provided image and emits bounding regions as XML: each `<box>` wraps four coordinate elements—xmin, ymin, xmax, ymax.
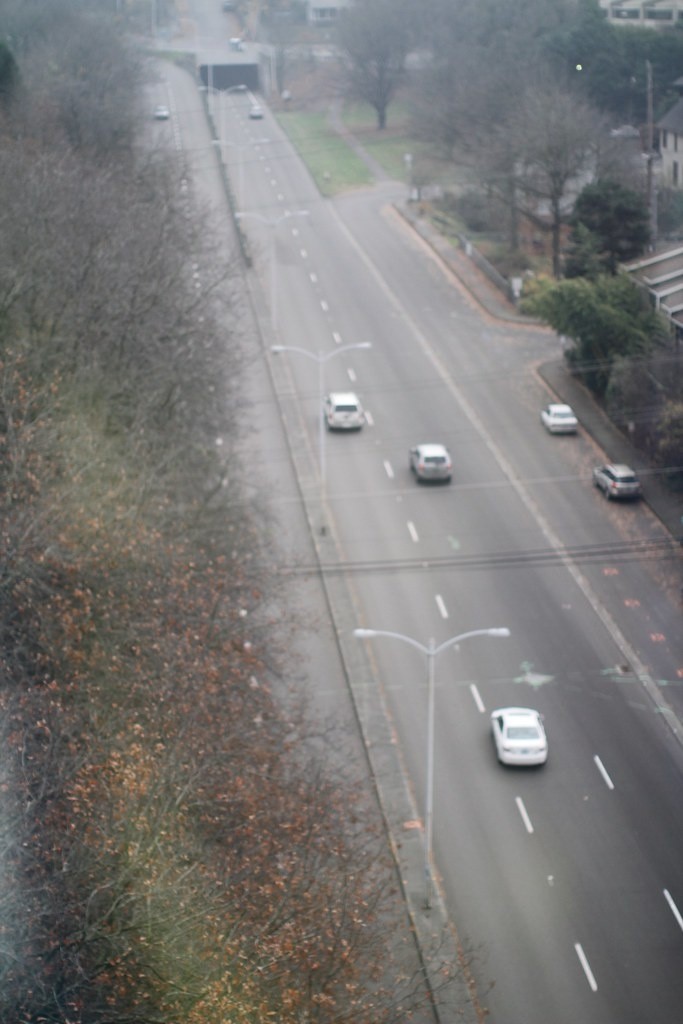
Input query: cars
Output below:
<box><xmin>154</xmin><ymin>105</ymin><xmax>169</xmax><ymax>120</ymax></box>
<box><xmin>492</xmin><ymin>707</ymin><xmax>546</xmax><ymax>767</ymax></box>
<box><xmin>328</xmin><ymin>393</ymin><xmax>363</xmax><ymax>433</ymax></box>
<box><xmin>249</xmin><ymin>106</ymin><xmax>263</xmax><ymax>119</ymax></box>
<box><xmin>541</xmin><ymin>405</ymin><xmax>576</xmax><ymax>431</ymax></box>
<box><xmin>592</xmin><ymin>463</ymin><xmax>642</xmax><ymax>498</ymax></box>
<box><xmin>411</xmin><ymin>443</ymin><xmax>451</xmax><ymax>483</ymax></box>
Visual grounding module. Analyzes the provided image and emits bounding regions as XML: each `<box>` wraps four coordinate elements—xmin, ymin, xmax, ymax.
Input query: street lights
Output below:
<box><xmin>195</xmin><ymin>83</ymin><xmax>247</xmax><ymax>134</ymax></box>
<box><xmin>272</xmin><ymin>342</ymin><xmax>372</xmax><ymax>511</ymax></box>
<box><xmin>353</xmin><ymin>627</ymin><xmax>511</xmax><ymax>863</ymax></box>
<box><xmin>210</xmin><ymin>137</ymin><xmax>264</xmax><ymax>207</ymax></box>
<box><xmin>231</xmin><ymin>210</ymin><xmax>311</xmax><ymax>331</ymax></box>
